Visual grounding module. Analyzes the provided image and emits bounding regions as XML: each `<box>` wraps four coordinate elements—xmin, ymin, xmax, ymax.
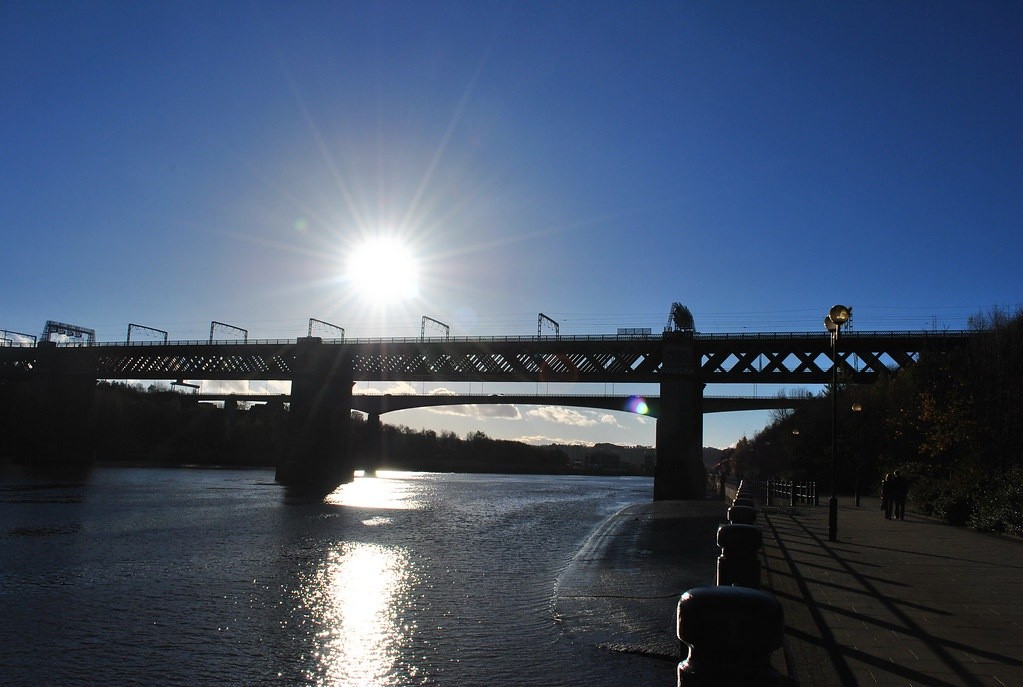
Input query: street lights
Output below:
<box><xmin>821</xmin><ymin>302</ymin><xmax>856</xmax><ymax>545</ymax></box>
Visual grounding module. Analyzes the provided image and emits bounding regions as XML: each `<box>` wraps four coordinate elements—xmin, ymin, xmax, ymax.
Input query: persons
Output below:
<box><xmin>881</xmin><ymin>471</ymin><xmax>909</xmax><ymax>520</ymax></box>
